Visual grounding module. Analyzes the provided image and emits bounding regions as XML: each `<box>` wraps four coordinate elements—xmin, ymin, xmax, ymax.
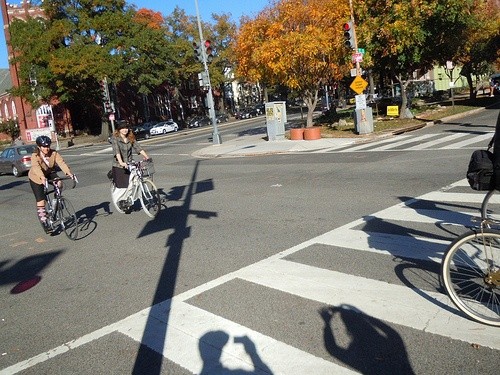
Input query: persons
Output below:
<box><xmin>111</xmin><ymin>122</ymin><xmax>150</xmax><ymax>204</ymax></box>
<box><xmin>28</xmin><ymin>136</ymin><xmax>75</xmax><ymax>228</ymax></box>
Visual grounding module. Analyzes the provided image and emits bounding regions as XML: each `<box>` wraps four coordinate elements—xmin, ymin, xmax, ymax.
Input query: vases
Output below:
<box><xmin>290</xmin><ymin>127</ymin><xmax>321</xmax><ymax>140</ymax></box>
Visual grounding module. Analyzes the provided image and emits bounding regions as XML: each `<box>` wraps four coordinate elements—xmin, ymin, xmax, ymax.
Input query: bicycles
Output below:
<box><xmin>480</xmin><ymin>187</ymin><xmax>500</xmax><ymax>245</ymax></box>
<box><xmin>37</xmin><ymin>173</ymin><xmax>79</xmax><ymax>241</ymax></box>
<box><xmin>441</xmin><ymin>216</ymin><xmax>500</xmax><ymax>328</ymax></box>
<box><xmin>110</xmin><ymin>159</ymin><xmax>161</xmax><ymax>219</ymax></box>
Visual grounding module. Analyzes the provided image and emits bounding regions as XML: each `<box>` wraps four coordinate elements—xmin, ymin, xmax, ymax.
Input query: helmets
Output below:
<box><xmin>36</xmin><ymin>136</ymin><xmax>52</xmax><ymax>146</ymax></box>
<box><xmin>117</xmin><ymin>121</ymin><xmax>129</xmax><ymax>131</ymax></box>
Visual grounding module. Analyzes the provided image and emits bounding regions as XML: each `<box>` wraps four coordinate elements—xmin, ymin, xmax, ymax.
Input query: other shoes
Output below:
<box><xmin>121</xmin><ymin>201</ymin><xmax>128</xmax><ymax>207</ymax></box>
<box><xmin>46</xmin><ymin>226</ymin><xmax>52</xmax><ymax>233</ymax></box>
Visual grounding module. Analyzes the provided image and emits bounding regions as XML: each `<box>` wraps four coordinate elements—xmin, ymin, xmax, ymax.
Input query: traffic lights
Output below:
<box><xmin>203</xmin><ymin>39</ymin><xmax>213</xmax><ymax>64</ymax></box>
<box><xmin>103</xmin><ymin>101</ymin><xmax>113</xmax><ymax>113</ymax></box>
<box><xmin>98</xmin><ymin>78</ymin><xmax>108</xmax><ymax>102</ymax></box>
<box><xmin>193</xmin><ymin>41</ymin><xmax>204</xmax><ymax>64</ymax></box>
<box><xmin>342</xmin><ymin>22</ymin><xmax>355</xmax><ymax>49</ymax></box>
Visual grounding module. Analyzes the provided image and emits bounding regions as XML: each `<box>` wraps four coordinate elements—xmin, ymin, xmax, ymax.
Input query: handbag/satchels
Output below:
<box><xmin>466</xmin><ymin>149</ymin><xmax>496</xmax><ymax>190</ymax></box>
<box><xmin>140</xmin><ymin>160</ymin><xmax>155</xmax><ymax>177</ymax></box>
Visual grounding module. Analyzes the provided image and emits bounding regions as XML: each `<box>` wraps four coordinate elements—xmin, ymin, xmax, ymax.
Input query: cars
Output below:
<box><xmin>108</xmin><ymin>125</ymin><xmax>151</xmax><ymax>144</ymax></box>
<box><xmin>0</xmin><ymin>143</ymin><xmax>38</xmax><ymax>177</ymax></box>
<box><xmin>234</xmin><ymin>103</ymin><xmax>265</xmax><ymax>120</ymax></box>
<box><xmin>150</xmin><ymin>121</ymin><xmax>179</xmax><ymax>137</ymax></box>
<box><xmin>186</xmin><ymin>113</ymin><xmax>229</xmax><ymax>129</ymax></box>
